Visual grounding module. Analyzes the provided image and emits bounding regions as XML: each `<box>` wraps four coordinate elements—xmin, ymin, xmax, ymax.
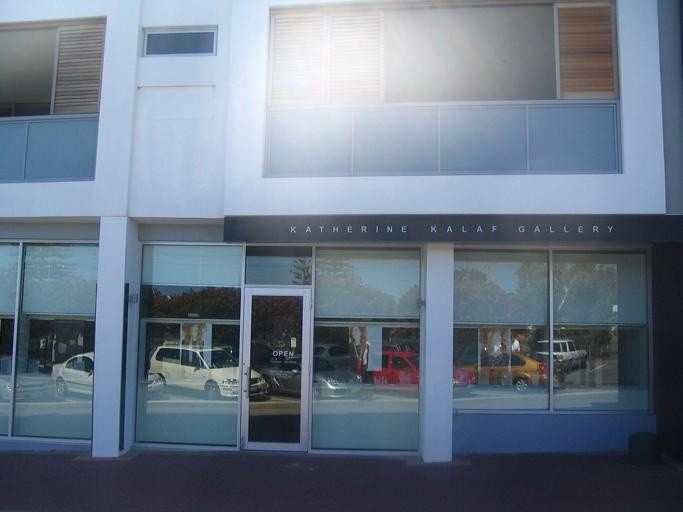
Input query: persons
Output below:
<box><xmin>350</xmin><ymin>324</ymin><xmax>369</xmax><ymax>368</ymax></box>
<box><xmin>493</xmin><ymin>338</ymin><xmax>505</xmax><ymax>352</ymax></box>
<box><xmin>512</xmin><ymin>336</ymin><xmax>521</xmax><ymax>352</ymax></box>
<box><xmin>181</xmin><ymin>320</ymin><xmax>207</xmax><ymax>367</ymax></box>
